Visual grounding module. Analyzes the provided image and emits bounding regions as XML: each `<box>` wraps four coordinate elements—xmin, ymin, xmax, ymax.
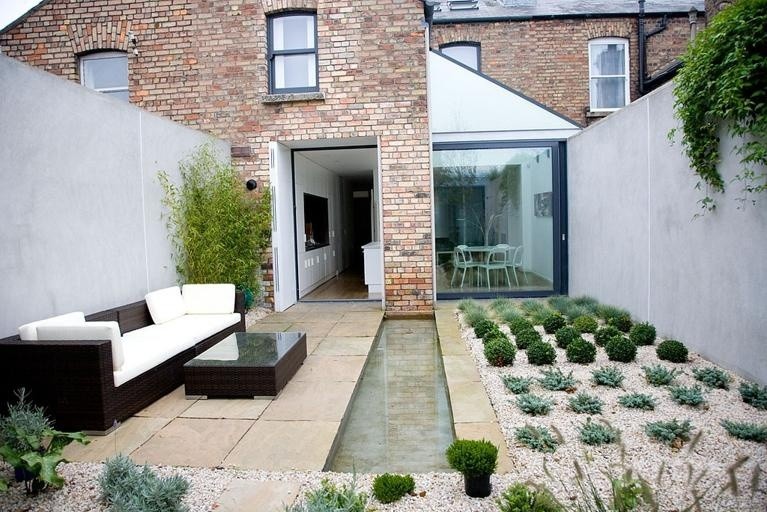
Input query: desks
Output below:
<box><xmin>181</xmin><ymin>329</ymin><xmax>308</xmax><ymax>400</ymax></box>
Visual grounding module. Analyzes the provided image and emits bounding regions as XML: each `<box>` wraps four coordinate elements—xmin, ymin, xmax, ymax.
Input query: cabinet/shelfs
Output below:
<box><xmin>292</xmin><ymin>151</ymin><xmax>350</xmax><ymax>297</ymax></box>
<box><xmin>361</xmin><ymin>241</ymin><xmax>384</xmax><ymax>295</ymax></box>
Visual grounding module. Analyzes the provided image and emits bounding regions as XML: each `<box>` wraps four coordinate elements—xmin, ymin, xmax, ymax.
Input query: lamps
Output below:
<box><xmin>246</xmin><ymin>179</ymin><xmax>257</xmax><ymax>191</ymax></box>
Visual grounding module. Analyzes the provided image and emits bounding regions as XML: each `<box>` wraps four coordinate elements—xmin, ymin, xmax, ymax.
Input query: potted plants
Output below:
<box><xmin>445</xmin><ymin>437</ymin><xmax>496</xmax><ymax>499</ymax></box>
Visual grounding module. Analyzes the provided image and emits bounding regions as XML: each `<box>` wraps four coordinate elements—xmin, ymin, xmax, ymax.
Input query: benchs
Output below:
<box><xmin>0</xmin><ymin>279</ymin><xmax>248</xmax><ymax>437</ymax></box>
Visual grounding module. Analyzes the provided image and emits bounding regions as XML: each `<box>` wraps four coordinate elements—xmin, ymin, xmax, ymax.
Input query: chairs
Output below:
<box><xmin>448</xmin><ymin>238</ymin><xmax>528</xmax><ymax>291</ymax></box>
